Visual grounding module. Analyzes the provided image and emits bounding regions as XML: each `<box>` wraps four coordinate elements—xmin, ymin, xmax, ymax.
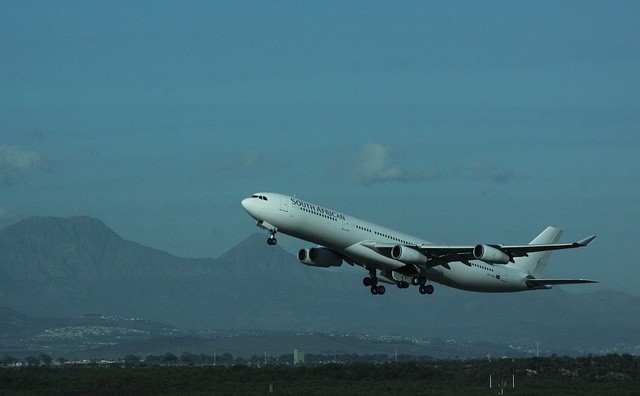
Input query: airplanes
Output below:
<box><xmin>241</xmin><ymin>191</ymin><xmax>600</xmax><ymax>294</ymax></box>
